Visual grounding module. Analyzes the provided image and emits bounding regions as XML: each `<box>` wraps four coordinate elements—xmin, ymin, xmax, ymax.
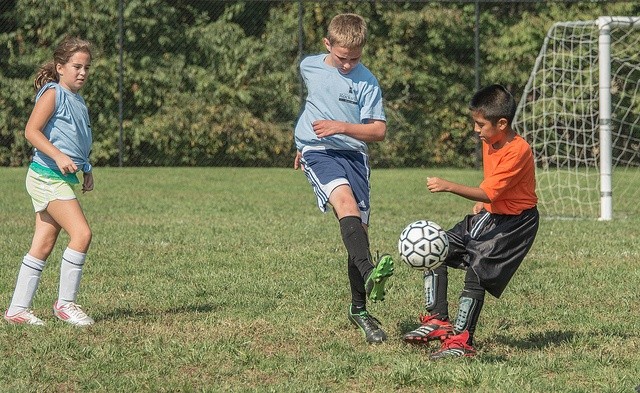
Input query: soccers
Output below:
<box><xmin>398</xmin><ymin>221</ymin><xmax>449</xmax><ymax>271</ymax></box>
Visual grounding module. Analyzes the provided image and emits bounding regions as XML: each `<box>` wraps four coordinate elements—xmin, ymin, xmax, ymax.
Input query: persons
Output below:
<box><xmin>2</xmin><ymin>34</ymin><xmax>96</xmax><ymax>325</ymax></box>
<box><xmin>293</xmin><ymin>13</ymin><xmax>395</xmax><ymax>347</ymax></box>
<box><xmin>401</xmin><ymin>84</ymin><xmax>541</xmax><ymax>361</ymax></box>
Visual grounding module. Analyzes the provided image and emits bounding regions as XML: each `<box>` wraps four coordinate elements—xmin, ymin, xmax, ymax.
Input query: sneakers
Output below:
<box><xmin>53</xmin><ymin>300</ymin><xmax>95</xmax><ymax>326</ymax></box>
<box><xmin>4</xmin><ymin>309</ymin><xmax>46</xmax><ymax>326</ymax></box>
<box><xmin>348</xmin><ymin>303</ymin><xmax>387</xmax><ymax>345</ymax></box>
<box><xmin>403</xmin><ymin>312</ymin><xmax>456</xmax><ymax>343</ymax></box>
<box><xmin>364</xmin><ymin>250</ymin><xmax>394</xmax><ymax>303</ymax></box>
<box><xmin>429</xmin><ymin>330</ymin><xmax>477</xmax><ymax>362</ymax></box>
